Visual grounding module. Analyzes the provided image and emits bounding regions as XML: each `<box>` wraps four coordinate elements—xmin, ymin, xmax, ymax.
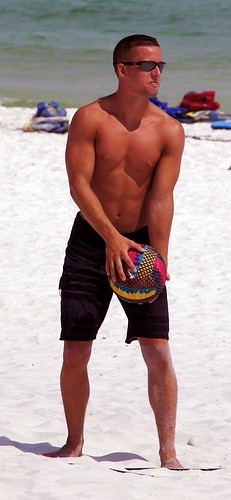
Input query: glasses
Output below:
<box><xmin>123</xmin><ymin>60</ymin><xmax>166</xmax><ymax>74</ymax></box>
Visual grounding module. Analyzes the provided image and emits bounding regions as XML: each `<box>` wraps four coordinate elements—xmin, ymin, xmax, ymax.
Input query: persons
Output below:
<box><xmin>42</xmin><ymin>34</ymin><xmax>186</xmax><ymax>470</ymax></box>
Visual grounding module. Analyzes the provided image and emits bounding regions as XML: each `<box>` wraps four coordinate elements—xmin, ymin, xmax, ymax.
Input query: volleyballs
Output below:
<box><xmin>108</xmin><ymin>244</ymin><xmax>167</xmax><ymax>303</ymax></box>
